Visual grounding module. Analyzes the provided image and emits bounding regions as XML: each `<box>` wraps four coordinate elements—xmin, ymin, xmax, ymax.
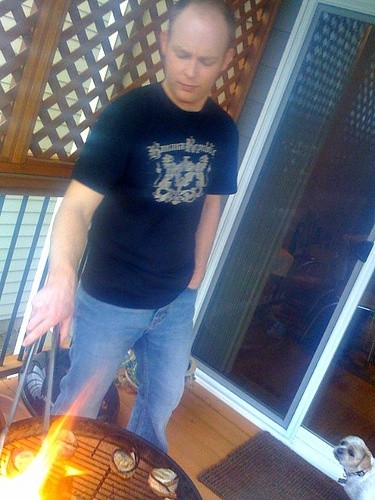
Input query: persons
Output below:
<box><xmin>19</xmin><ymin>0</ymin><xmax>242</xmax><ymax>455</ymax></box>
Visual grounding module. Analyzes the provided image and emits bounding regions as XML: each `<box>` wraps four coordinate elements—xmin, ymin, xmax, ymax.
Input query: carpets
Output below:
<box><xmin>197</xmin><ymin>431</ymin><xmax>350</xmax><ymax>500</ymax></box>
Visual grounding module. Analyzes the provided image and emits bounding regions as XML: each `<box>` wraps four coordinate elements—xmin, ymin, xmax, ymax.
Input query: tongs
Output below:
<box><xmin>0</xmin><ymin>322</ymin><xmax>60</xmax><ymax>459</ymax></box>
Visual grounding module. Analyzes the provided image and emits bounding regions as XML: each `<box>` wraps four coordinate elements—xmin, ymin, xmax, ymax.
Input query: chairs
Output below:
<box><xmin>299</xmin><ymin>286</ymin><xmax>375</xmax><ymax>368</ymax></box>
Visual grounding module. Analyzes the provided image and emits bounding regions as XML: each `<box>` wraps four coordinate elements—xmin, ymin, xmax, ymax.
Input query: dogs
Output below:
<box><xmin>333</xmin><ymin>435</ymin><xmax>375</xmax><ymax>500</ymax></box>
